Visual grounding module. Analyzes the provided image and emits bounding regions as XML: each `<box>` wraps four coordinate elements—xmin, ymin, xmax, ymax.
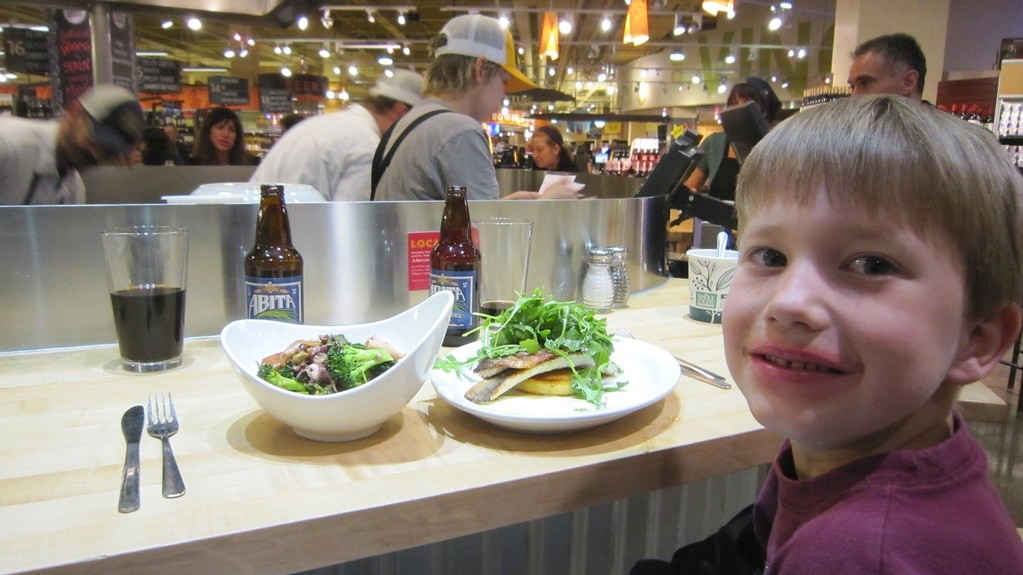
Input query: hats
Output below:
<box><xmin>745</xmin><ymin>76</ymin><xmax>782</xmax><ymax>117</ymax></box>
<box><xmin>434</xmin><ymin>14</ymin><xmax>539</xmax><ymax>93</ymax></box>
<box><xmin>79</xmin><ymin>84</ymin><xmax>144</xmax><ymax>156</ymax></box>
<box><xmin>539</xmin><ymin>126</ymin><xmax>563</xmax><ymax>147</ymax></box>
<box><xmin>369</xmin><ymin>70</ymin><xmax>425</xmax><ymax>107</ymax></box>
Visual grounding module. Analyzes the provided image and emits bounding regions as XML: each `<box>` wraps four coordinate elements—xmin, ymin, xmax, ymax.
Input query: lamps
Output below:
<box><xmin>669</xmin><ymin>17</ymin><xmax>737</xmax><ymax>94</ymax></box>
<box><xmin>538</xmin><ymin>0</ymin><xmax>560</xmax><ymax>62</ymax></box>
<box><xmin>701</xmin><ymin>0</ymin><xmax>736</xmax><ymax>19</ymax></box>
<box><xmin>318</xmin><ymin>6</ymin><xmax>417</xmax><ymax>29</ymax></box>
<box><xmin>622</xmin><ymin>0</ymin><xmax>650</xmax><ymax>47</ymax></box>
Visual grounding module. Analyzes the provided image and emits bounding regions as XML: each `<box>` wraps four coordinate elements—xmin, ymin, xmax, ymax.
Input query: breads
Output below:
<box><xmin>514</xmin><ymin>376</ymin><xmax>578</xmax><ymax>395</ymax></box>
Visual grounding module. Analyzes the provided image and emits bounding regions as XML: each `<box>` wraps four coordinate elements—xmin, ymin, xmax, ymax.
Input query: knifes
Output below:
<box><xmin>118</xmin><ymin>406</ymin><xmax>145</xmax><ymax>513</ymax></box>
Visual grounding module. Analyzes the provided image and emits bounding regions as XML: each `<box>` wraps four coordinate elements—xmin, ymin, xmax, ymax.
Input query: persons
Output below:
<box><xmin>0</xmin><ymin>82</ymin><xmax>190</xmax><ymax>205</ymax></box>
<box><xmin>531</xmin><ymin>126</ymin><xmax>581</xmax><ymax>172</ymax></box>
<box><xmin>628</xmin><ymin>92</ymin><xmax>1023</xmax><ymax>575</ymax></box>
<box><xmin>279</xmin><ymin>113</ymin><xmax>305</xmax><ymax>134</ymax></box>
<box><xmin>191</xmin><ymin>107</ymin><xmax>260</xmax><ymax>166</ymax></box>
<box><xmin>248</xmin><ymin>68</ymin><xmax>426</xmax><ymax>202</ymax></box>
<box><xmin>369</xmin><ymin>14</ymin><xmax>541</xmax><ymax>201</ymax></box>
<box><xmin>847</xmin><ymin>32</ymin><xmax>926</xmax><ymax>101</ymax></box>
<box><xmin>683</xmin><ymin>76</ymin><xmax>782</xmax><ymax>203</ymax></box>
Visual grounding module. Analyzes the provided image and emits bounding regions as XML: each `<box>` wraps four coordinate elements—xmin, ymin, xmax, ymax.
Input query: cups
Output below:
<box><xmin>469</xmin><ymin>215</ymin><xmax>540</xmax><ymax>321</ymax></box>
<box><xmin>685</xmin><ymin>248</ymin><xmax>740</xmax><ymax>323</ymax></box>
<box><xmin>100</xmin><ymin>225</ymin><xmax>192</xmax><ymax>375</ymax></box>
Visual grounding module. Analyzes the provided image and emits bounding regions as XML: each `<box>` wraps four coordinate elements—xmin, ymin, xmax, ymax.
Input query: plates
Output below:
<box><xmin>431</xmin><ymin>333</ymin><xmax>682</xmax><ymax>436</ymax></box>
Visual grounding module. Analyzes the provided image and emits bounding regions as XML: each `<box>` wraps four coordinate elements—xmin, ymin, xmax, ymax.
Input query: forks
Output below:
<box><xmin>146</xmin><ymin>391</ymin><xmax>186</xmax><ymax>499</ymax></box>
<box><xmin>609</xmin><ymin>326</ymin><xmax>728</xmax><ymax>381</ymax></box>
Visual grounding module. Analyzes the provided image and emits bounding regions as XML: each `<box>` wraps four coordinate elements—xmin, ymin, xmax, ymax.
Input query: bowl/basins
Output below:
<box><xmin>219</xmin><ymin>290</ymin><xmax>455</xmax><ymax>443</ymax></box>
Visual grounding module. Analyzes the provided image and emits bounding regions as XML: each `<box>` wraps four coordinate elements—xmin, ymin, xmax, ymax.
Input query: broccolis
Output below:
<box><xmin>256</xmin><ymin>333</ymin><xmax>399</xmax><ymax>396</ymax></box>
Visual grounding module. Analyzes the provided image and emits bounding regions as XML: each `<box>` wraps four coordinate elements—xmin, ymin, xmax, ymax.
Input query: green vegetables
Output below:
<box><xmin>433</xmin><ymin>288</ymin><xmax>630</xmax><ymax>409</ymax></box>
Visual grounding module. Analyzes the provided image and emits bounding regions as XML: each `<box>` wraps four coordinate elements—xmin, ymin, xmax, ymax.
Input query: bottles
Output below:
<box><xmin>800</xmin><ymin>85</ymin><xmax>852</xmax><ymax>113</ymax></box>
<box><xmin>550</xmin><ymin>238</ymin><xmax>599</xmax><ymax>303</ymax></box>
<box><xmin>428</xmin><ymin>185</ymin><xmax>483</xmax><ymax>348</ymax></box>
<box><xmin>580</xmin><ymin>245</ymin><xmax>633</xmax><ymax>314</ymax></box>
<box><xmin>934</xmin><ymin>103</ymin><xmax>993</xmax><ymax>133</ymax></box>
<box><xmin>24</xmin><ymin>95</ymin><xmax>52</xmax><ymax>119</ymax></box>
<box><xmin>243</xmin><ymin>185</ymin><xmax>303</xmax><ymax>326</ymax></box>
<box><xmin>631</xmin><ymin>146</ymin><xmax>663</xmax><ymax>178</ymax></box>
